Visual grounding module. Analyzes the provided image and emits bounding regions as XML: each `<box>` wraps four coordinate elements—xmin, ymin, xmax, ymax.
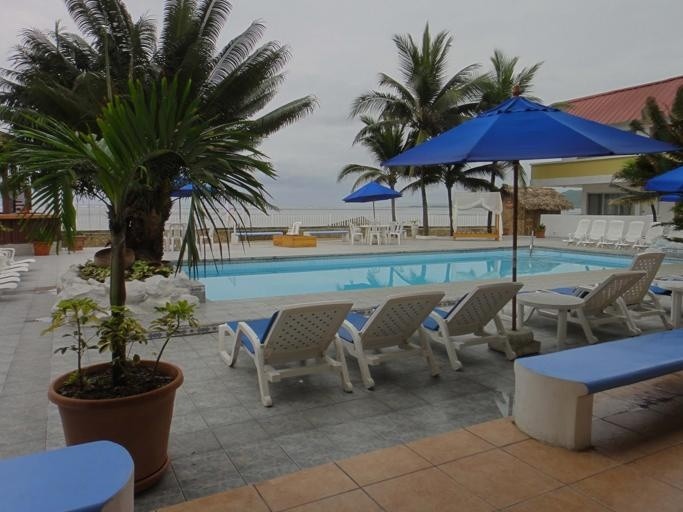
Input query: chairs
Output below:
<box><xmin>1</xmin><ymin>248</ymin><xmax>36</xmax><ymax>293</ymax></box>
<box><xmin>0</xmin><ymin>439</ymin><xmax>135</xmax><ymax>512</ymax></box>
<box><xmin>562</xmin><ymin>219</ymin><xmax>683</xmax><ymax>256</ymax></box>
<box><xmin>163</xmin><ymin>220</ymin><xmax>215</xmax><ymax>253</ymax></box>
<box><xmin>347</xmin><ymin>220</ymin><xmax>406</xmax><ymax>246</ymax></box>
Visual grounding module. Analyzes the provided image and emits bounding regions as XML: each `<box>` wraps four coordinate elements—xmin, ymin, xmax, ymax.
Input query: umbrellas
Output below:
<box><xmin>165</xmin><ymin>171</ymin><xmax>213</xmax><ymax>222</ymax></box>
<box><xmin>337</xmin><ymin>180</ymin><xmax>403</xmax><ymax>218</ymax></box>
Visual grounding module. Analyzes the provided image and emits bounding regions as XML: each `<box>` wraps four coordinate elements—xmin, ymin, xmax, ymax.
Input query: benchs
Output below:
<box><xmin>230</xmin><ymin>232</ymin><xmax>283</xmax><ymax>244</ymax></box>
<box><xmin>303</xmin><ymin>231</ymin><xmax>349</xmax><ymax>242</ymax></box>
<box><xmin>512</xmin><ymin>328</ymin><xmax>683</xmax><ymax>451</ymax></box>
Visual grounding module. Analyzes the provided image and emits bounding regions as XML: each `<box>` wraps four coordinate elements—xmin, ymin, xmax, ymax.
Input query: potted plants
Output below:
<box><xmin>534</xmin><ymin>222</ymin><xmax>546</xmax><ymax>238</ymax></box>
<box><xmin>1</xmin><ymin>71</ymin><xmax>280</xmax><ymax>496</ymax></box>
<box><xmin>26</xmin><ymin>225</ymin><xmax>57</xmax><ymax>256</ymax></box>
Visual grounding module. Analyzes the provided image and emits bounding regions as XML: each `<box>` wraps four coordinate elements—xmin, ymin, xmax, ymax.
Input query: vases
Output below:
<box><xmin>71</xmin><ymin>235</ymin><xmax>89</xmax><ymax>250</ymax></box>
